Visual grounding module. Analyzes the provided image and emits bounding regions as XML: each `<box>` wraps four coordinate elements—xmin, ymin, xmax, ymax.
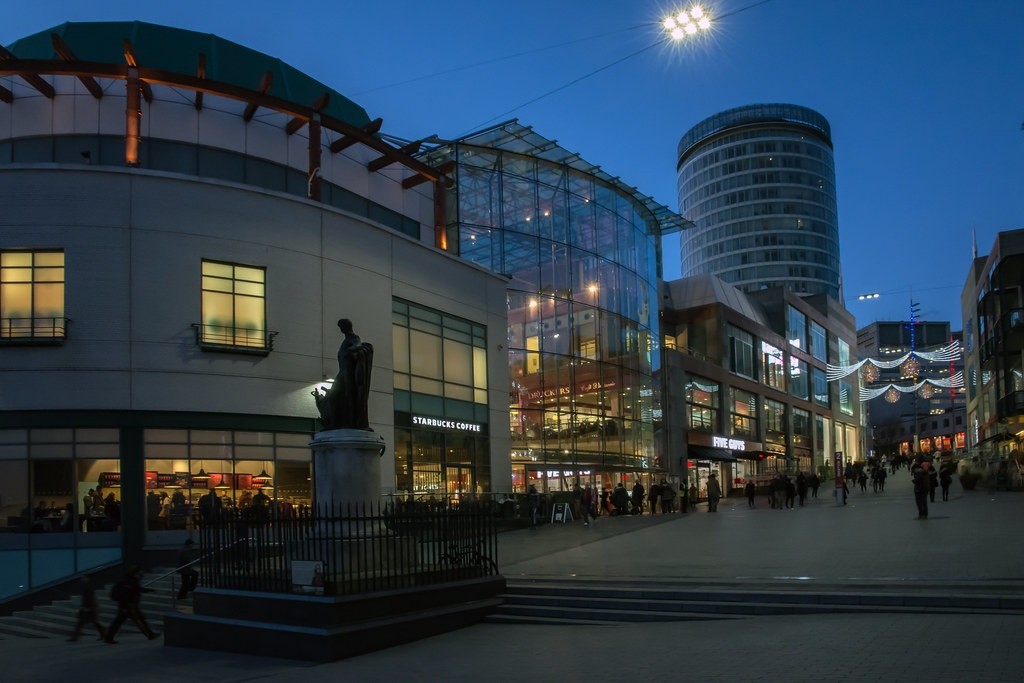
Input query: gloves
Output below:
<box><xmin>147</xmin><ymin>588</ymin><xmax>155</xmax><ymax>592</ymax></box>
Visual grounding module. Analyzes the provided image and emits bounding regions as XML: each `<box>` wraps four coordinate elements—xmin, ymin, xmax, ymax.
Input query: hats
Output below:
<box><xmin>635</xmin><ymin>480</ymin><xmax>640</xmax><ymax>483</ymax></box>
<box><xmin>710</xmin><ymin>474</ymin><xmax>716</xmax><ymax>477</ymax></box>
<box><xmin>184</xmin><ymin>539</ymin><xmax>194</xmax><ymax>546</ymax></box>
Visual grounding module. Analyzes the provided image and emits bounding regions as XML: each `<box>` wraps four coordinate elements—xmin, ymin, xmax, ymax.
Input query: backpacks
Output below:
<box><xmin>175</xmin><ymin>546</ymin><xmax>190</xmax><ymax>563</ymax></box>
<box><xmin>108</xmin><ymin>576</ymin><xmax>137</xmax><ymax>602</ymax></box>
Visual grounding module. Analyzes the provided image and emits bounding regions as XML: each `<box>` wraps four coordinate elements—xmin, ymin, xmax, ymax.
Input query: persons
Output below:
<box><xmin>744</xmin><ymin>480</ymin><xmax>755</xmax><ymax>507</ymax></box>
<box><xmin>528</xmin><ymin>484</ymin><xmax>540</xmax><ymax>530</ymax></box>
<box><xmin>33</xmin><ymin>485</ymin><xmax>294</xmax><ymax>532</ymax></box>
<box><xmin>329</xmin><ymin>319</ymin><xmax>373</xmax><ymax>428</ymax></box>
<box><xmin>911</xmin><ymin>464</ymin><xmax>930</xmax><ymax>520</ymax></box>
<box><xmin>573</xmin><ymin>474</ymin><xmax>721</xmax><ymax>525</ymax></box>
<box><xmin>767</xmin><ymin>449</ymin><xmax>1006</xmax><ymax>509</ymax></box>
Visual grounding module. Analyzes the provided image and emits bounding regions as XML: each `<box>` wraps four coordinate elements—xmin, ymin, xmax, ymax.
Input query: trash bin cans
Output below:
<box><xmin>1016</xmin><ymin>473</ymin><xmax>1024</xmax><ymax>487</ymax></box>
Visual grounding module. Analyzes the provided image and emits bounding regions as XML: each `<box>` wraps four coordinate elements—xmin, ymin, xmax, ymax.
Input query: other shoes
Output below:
<box><xmin>103</xmin><ymin>639</ymin><xmax>119</xmax><ymax>644</ymax></box>
<box><xmin>187</xmin><ymin>591</ymin><xmax>193</xmax><ymax>598</ymax></box>
<box><xmin>64</xmin><ymin>638</ymin><xmax>77</xmax><ymax>644</ymax></box>
<box><xmin>96</xmin><ymin>637</ymin><xmax>105</xmax><ymax>643</ymax></box>
<box><xmin>584</xmin><ymin>522</ymin><xmax>589</xmax><ymax>526</ymax></box>
<box><xmin>593</xmin><ymin>519</ymin><xmax>598</xmax><ymax>524</ymax></box>
<box><xmin>791</xmin><ymin>507</ymin><xmax>794</xmax><ymax>511</ymax></box>
<box><xmin>148</xmin><ymin>633</ymin><xmax>162</xmax><ymax>640</ymax></box>
<box><xmin>177</xmin><ymin>592</ymin><xmax>185</xmax><ymax>599</ymax></box>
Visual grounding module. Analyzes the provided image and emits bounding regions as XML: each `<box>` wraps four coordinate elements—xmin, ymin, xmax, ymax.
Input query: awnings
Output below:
<box><xmin>973</xmin><ymin>432</ymin><xmax>1019</xmax><ymax>447</ymax></box>
